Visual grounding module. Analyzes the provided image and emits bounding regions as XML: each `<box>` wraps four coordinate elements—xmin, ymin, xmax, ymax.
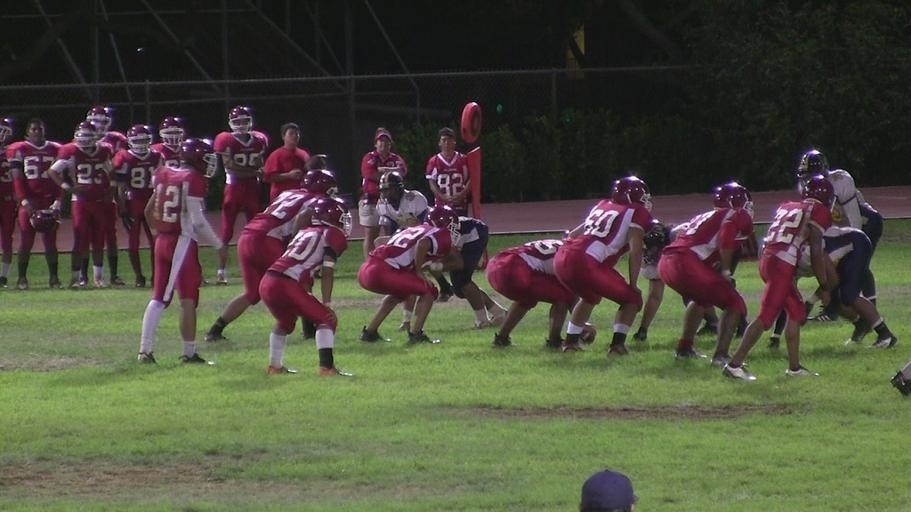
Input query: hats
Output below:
<box><xmin>373</xmin><ymin>127</ymin><xmax>394</xmax><ymax>145</ymax></box>
<box><xmin>438</xmin><ymin>128</ymin><xmax>456</xmax><ymax>142</ymax></box>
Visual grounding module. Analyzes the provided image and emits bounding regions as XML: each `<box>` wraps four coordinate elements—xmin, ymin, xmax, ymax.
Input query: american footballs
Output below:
<box><xmin>580</xmin><ymin>330</ymin><xmax>595</xmax><ymax>343</ymax></box>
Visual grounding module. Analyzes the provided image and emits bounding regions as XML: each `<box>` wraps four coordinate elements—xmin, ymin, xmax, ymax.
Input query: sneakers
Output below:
<box><xmin>137</xmin><ymin>321</ymin><xmax>440</xmax><ymax>378</ymax></box>
<box><xmin>471</xmin><ymin>299</ymin><xmax>898</xmax><ymax>379</ymax></box>
<box><xmin>437</xmin><ymin>286</ymin><xmax>454</xmax><ymax>303</ymax></box>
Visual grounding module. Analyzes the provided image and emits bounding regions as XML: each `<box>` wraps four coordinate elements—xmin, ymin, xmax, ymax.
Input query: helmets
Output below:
<box><xmin>611</xmin><ymin>149</ymin><xmax>832</xmax><ymax>264</ymax></box>
<box><xmin>0</xmin><ymin>106</ymin><xmax>255</xmax><ymax>178</ymax></box>
<box><xmin>30</xmin><ymin>209</ymin><xmax>60</xmax><ymax>234</ymax></box>
<box><xmin>303</xmin><ymin>166</ymin><xmax>459</xmax><ymax>246</ymax></box>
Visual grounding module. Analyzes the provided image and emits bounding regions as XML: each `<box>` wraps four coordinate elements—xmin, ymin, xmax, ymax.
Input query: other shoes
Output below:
<box><xmin>892</xmin><ymin>371</ymin><xmax>910</xmax><ymax>397</ymax></box>
<box><xmin>0</xmin><ymin>269</ymin><xmax>229</xmax><ymax>290</ymax></box>
